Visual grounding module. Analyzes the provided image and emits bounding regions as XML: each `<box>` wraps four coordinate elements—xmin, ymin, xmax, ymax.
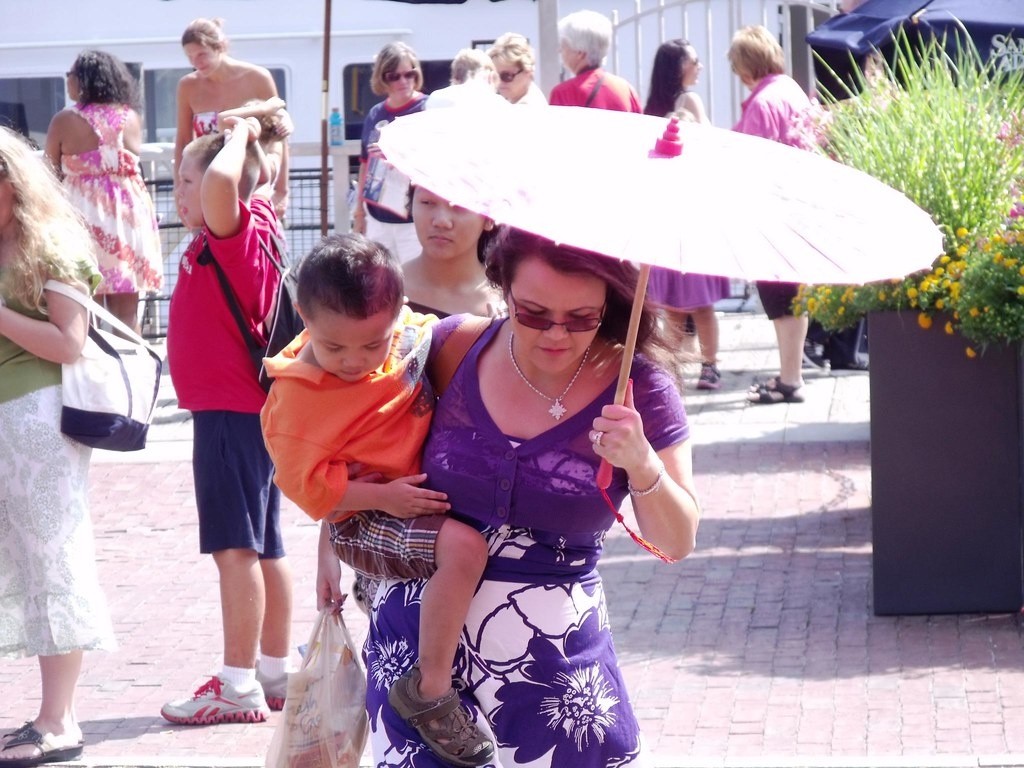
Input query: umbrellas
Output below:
<box><xmin>380</xmin><ymin>103</ymin><xmax>947</xmax><ymax>559</ymax></box>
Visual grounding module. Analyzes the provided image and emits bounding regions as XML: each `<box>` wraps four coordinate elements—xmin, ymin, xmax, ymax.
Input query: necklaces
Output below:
<box><xmin>510</xmin><ymin>330</ymin><xmax>590</xmax><ymax>420</ymax></box>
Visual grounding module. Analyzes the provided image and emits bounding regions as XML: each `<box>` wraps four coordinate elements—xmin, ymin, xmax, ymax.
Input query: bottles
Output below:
<box><xmin>328</xmin><ymin>107</ymin><xmax>345</xmax><ymax>144</ymax></box>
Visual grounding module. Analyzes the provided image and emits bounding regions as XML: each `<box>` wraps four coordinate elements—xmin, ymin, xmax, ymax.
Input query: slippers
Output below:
<box><xmin>0</xmin><ymin>721</ymin><xmax>85</xmax><ymax>767</ymax></box>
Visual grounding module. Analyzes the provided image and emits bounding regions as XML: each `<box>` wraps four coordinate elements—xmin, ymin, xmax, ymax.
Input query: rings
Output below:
<box><xmin>595</xmin><ymin>432</ymin><xmax>603</xmax><ymax>446</ymax></box>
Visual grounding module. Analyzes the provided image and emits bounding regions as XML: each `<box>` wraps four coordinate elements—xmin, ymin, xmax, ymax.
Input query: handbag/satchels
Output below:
<box><xmin>262</xmin><ymin>601</ymin><xmax>370</xmax><ymax>768</ymax></box>
<box><xmin>42</xmin><ymin>278</ymin><xmax>165</xmax><ymax>452</ymax></box>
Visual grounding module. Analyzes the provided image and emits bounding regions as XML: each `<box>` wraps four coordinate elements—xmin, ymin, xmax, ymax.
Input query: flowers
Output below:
<box><xmin>792</xmin><ymin>11</ymin><xmax>1024</xmax><ymax>358</ymax></box>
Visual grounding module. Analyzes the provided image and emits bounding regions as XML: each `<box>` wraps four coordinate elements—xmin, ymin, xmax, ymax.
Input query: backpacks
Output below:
<box><xmin>205</xmin><ymin>219</ymin><xmax>310</xmax><ymax>393</ymax></box>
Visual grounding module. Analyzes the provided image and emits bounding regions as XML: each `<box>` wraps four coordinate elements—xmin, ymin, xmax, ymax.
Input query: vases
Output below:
<box><xmin>867</xmin><ymin>309</ymin><xmax>1024</xmax><ymax>614</ymax></box>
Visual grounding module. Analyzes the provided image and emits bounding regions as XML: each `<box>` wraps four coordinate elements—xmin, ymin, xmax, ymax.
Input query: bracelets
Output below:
<box><xmin>354</xmin><ymin>211</ymin><xmax>366</xmax><ymax>218</ymax></box>
<box><xmin>628</xmin><ymin>466</ymin><xmax>664</xmax><ymax>497</ymax></box>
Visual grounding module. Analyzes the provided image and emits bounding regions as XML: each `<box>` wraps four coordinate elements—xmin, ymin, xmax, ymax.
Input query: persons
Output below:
<box><xmin>551</xmin><ymin>8</ymin><xmax>645</xmax><ymax>114</ymax></box>
<box><xmin>160</xmin><ymin>97</ymin><xmax>293</xmax><ymax>726</ymax></box>
<box><xmin>804</xmin><ymin>317</ymin><xmax>869</xmax><ymax>376</ymax></box>
<box><xmin>728</xmin><ymin>26</ymin><xmax>813</xmax><ymax>402</ymax></box>
<box><xmin>259</xmin><ymin>233</ymin><xmax>493</xmax><ymax>768</ymax></box>
<box><xmin>644</xmin><ymin>40</ymin><xmax>731</xmax><ymax>388</ymax></box>
<box><xmin>425</xmin><ymin>49</ymin><xmax>508</xmax><ymax>109</ymax></box>
<box><xmin>46</xmin><ymin>49</ymin><xmax>164</xmax><ymax>342</ymax></box>
<box><xmin>488</xmin><ymin>33</ymin><xmax>547</xmax><ymax>104</ymax></box>
<box><xmin>173</xmin><ymin>17</ymin><xmax>288</xmax><ymax>230</ymax></box>
<box><xmin>0</xmin><ymin>126</ymin><xmax>120</xmax><ymax>768</ymax></box>
<box><xmin>317</xmin><ymin>183</ymin><xmax>507</xmax><ymax>615</ymax></box>
<box><xmin>349</xmin><ymin>226</ymin><xmax>698</xmax><ymax>768</ymax></box>
<box><xmin>353</xmin><ymin>41</ymin><xmax>428</xmax><ymax>264</ymax></box>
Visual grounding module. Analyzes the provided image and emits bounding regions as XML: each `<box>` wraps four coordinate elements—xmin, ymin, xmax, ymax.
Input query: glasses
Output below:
<box><xmin>498</xmin><ymin>69</ymin><xmax>524</xmax><ymax>81</ymax></box>
<box><xmin>508</xmin><ymin>288</ymin><xmax>607</xmax><ymax>333</ymax></box>
<box><xmin>381</xmin><ymin>68</ymin><xmax>418</xmax><ymax>81</ymax></box>
<box><xmin>66</xmin><ymin>71</ymin><xmax>74</xmax><ymax>78</ymax></box>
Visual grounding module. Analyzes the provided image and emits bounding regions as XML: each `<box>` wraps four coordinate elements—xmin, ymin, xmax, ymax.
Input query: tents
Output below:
<box><xmin>806</xmin><ymin>0</ymin><xmax>1024</xmax><ymax>103</ymax></box>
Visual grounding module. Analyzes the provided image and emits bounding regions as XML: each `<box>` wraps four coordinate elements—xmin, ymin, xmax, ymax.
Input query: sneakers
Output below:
<box><xmin>255</xmin><ymin>657</ymin><xmax>290</xmax><ymax>710</ymax></box>
<box><xmin>160</xmin><ymin>673</ymin><xmax>271</xmax><ymax>725</ymax></box>
<box><xmin>802</xmin><ymin>338</ymin><xmax>828</xmax><ymax>373</ymax></box>
<box><xmin>829</xmin><ymin>359</ymin><xmax>870</xmax><ymax>376</ymax></box>
<box><xmin>697</xmin><ymin>363</ymin><xmax>722</xmax><ymax>389</ymax></box>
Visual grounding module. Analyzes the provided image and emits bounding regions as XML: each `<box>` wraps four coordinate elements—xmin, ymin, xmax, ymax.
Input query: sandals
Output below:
<box><xmin>745</xmin><ymin>376</ymin><xmax>805</xmax><ymax>404</ymax></box>
<box><xmin>387</xmin><ymin>663</ymin><xmax>495</xmax><ymax>767</ymax></box>
